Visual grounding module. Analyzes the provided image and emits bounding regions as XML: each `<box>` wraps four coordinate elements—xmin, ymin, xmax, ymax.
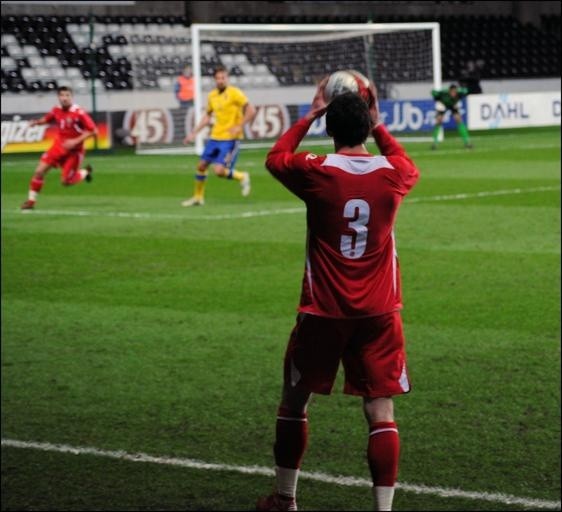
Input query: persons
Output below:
<box><xmin>431</xmin><ymin>84</ymin><xmax>474</xmax><ymax>150</ymax></box>
<box><xmin>181</xmin><ymin>66</ymin><xmax>255</xmax><ymax>207</ymax></box>
<box><xmin>174</xmin><ymin>66</ymin><xmax>194</xmax><ymax>108</ymax></box>
<box><xmin>459</xmin><ymin>62</ymin><xmax>481</xmax><ymax>93</ymax></box>
<box><xmin>19</xmin><ymin>87</ymin><xmax>99</xmax><ymax>209</ymax></box>
<box><xmin>255</xmin><ymin>77</ymin><xmax>420</xmax><ymax>512</ymax></box>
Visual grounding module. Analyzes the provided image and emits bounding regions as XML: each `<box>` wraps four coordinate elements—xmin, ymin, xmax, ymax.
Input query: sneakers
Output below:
<box><xmin>20</xmin><ymin>200</ymin><xmax>33</xmax><ymax>209</ymax></box>
<box><xmin>240</xmin><ymin>175</ymin><xmax>250</xmax><ymax>198</ymax></box>
<box><xmin>83</xmin><ymin>164</ymin><xmax>93</xmax><ymax>181</ymax></box>
<box><xmin>182</xmin><ymin>196</ymin><xmax>204</xmax><ymax>207</ymax></box>
<box><xmin>256</xmin><ymin>494</ymin><xmax>298</xmax><ymax>511</ymax></box>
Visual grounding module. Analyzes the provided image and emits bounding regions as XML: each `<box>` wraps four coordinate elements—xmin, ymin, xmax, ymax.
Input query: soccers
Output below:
<box><xmin>325</xmin><ymin>68</ymin><xmax>374</xmax><ymax>108</ymax></box>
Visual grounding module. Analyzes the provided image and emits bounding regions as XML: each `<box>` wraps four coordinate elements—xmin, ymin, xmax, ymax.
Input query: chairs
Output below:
<box><xmin>1</xmin><ymin>0</ymin><xmax>562</xmax><ymax>97</ymax></box>
